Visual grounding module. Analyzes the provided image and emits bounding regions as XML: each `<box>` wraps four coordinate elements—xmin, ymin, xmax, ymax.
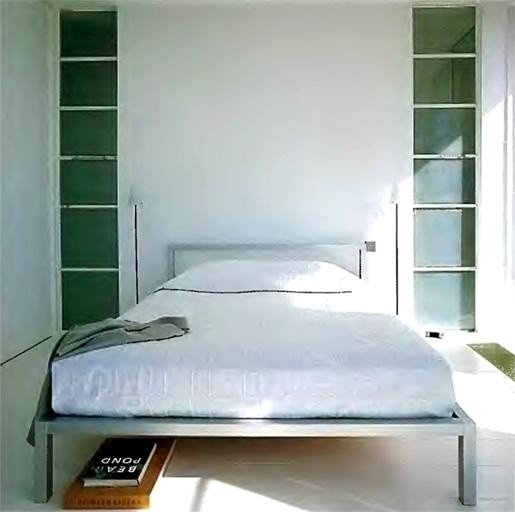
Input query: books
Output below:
<box><xmin>62</xmin><ymin>437</ymin><xmax>179</xmax><ymax>510</ymax></box>
<box><xmin>75</xmin><ymin>436</ymin><xmax>158</xmax><ymax>489</ymax></box>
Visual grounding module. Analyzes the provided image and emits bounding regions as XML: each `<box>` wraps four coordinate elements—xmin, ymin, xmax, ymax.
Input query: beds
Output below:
<box><xmin>33</xmin><ymin>240</ymin><xmax>478</xmax><ymax>506</ymax></box>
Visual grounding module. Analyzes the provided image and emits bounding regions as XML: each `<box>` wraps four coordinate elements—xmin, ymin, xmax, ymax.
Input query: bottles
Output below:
<box><xmin>426</xmin><ymin>331</ymin><xmax>446</xmax><ymax>339</ymax></box>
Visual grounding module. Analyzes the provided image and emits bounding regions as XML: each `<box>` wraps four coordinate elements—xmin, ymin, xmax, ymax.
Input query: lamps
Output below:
<box><xmin>128</xmin><ymin>184</ymin><xmax>146</xmax><ymax>306</ymax></box>
<box><xmin>390</xmin><ymin>183</ymin><xmax>403</xmax><ymax>316</ymax></box>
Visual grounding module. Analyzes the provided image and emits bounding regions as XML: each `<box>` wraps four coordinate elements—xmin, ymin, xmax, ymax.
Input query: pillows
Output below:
<box><xmin>146</xmin><ymin>260</ymin><xmax>373</xmax><ymax>293</ymax></box>
